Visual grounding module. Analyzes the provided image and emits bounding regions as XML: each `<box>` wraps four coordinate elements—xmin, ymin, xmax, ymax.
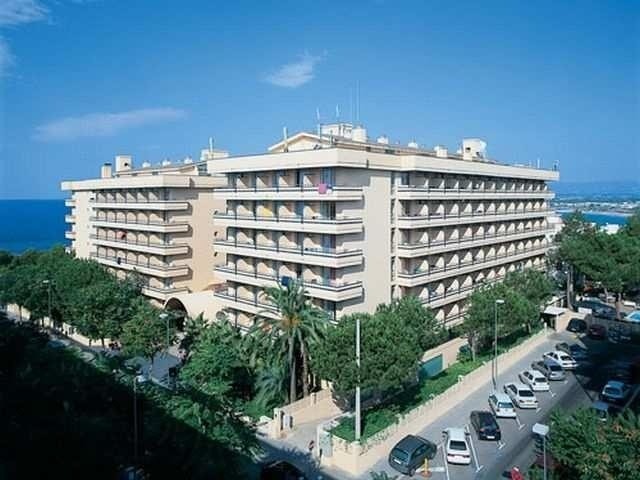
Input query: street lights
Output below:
<box><xmin>493</xmin><ymin>299</ymin><xmax>505</xmax><ymax>388</ymax></box>
<box><xmin>595</xmin><ymin>280</ymin><xmax>608</xmax><ymax>304</ymax></box>
<box><xmin>42</xmin><ymin>278</ymin><xmax>54</xmax><ymax>331</ymax></box>
<box><xmin>565</xmin><ymin>271</ymin><xmax>570</xmax><ymax>307</ymax></box>
<box><xmin>126</xmin><ymin>365</ymin><xmax>143</xmax><ymax>462</ymax></box>
<box><xmin>532</xmin><ymin>422</ymin><xmax>549</xmax><ymax>480</ymax></box>
<box><xmin>157</xmin><ymin>309</ymin><xmax>184</xmax><ymax>388</ymax></box>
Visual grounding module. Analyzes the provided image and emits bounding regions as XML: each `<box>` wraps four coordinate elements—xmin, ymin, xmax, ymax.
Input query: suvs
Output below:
<box><xmin>388</xmin><ymin>434</ymin><xmax>437</xmax><ymax>477</ymax></box>
<box><xmin>261</xmin><ymin>460</ymin><xmax>308</xmax><ymax>480</ymax></box>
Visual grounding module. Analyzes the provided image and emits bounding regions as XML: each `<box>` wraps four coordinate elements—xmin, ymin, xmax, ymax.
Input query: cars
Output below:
<box><xmin>572</xmin><ymin>299</ymin><xmax>639</xmax><ymax>323</ymax></box>
<box><xmin>518</xmin><ymin>370</ymin><xmax>550</xmax><ymax>393</ymax></box>
<box><xmin>531</xmin><ymin>360</ymin><xmax>565</xmax><ymax>381</ymax></box>
<box><xmin>488</xmin><ymin>394</ymin><xmax>516</xmax><ymax>419</ymax></box>
<box><xmin>575</xmin><ymin>402</ymin><xmax>614</xmax><ymax>430</ymax></box>
<box><xmin>504</xmin><ymin>383</ymin><xmax>539</xmax><ymax>409</ymax></box>
<box><xmin>531</xmin><ymin>454</ymin><xmax>561</xmax><ymax>472</ymax></box>
<box><xmin>471</xmin><ymin>409</ymin><xmax>502</xmax><ymax>442</ymax></box>
<box><xmin>587</xmin><ymin>324</ymin><xmax>605</xmax><ymax>339</ymax></box>
<box><xmin>544</xmin><ymin>351</ymin><xmax>577</xmax><ymax>371</ymax></box>
<box><xmin>583</xmin><ymin>287</ymin><xmax>604</xmax><ymax>298</ymax></box>
<box><xmin>442</xmin><ymin>427</ymin><xmax>471</xmax><ymax>466</ymax></box>
<box><xmin>599</xmin><ymin>379</ymin><xmax>632</xmax><ymax>404</ymax></box>
<box><xmin>567</xmin><ymin>319</ymin><xmax>587</xmax><ymax>333</ymax></box>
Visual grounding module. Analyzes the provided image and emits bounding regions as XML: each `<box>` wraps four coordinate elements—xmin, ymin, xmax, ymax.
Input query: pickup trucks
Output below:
<box><xmin>555</xmin><ymin>342</ymin><xmax>589</xmax><ymax>360</ymax></box>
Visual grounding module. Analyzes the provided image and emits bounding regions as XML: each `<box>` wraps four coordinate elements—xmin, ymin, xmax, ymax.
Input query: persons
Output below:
<box><xmin>308</xmin><ymin>439</ymin><xmax>315</xmax><ymax>452</ymax></box>
<box><xmin>511</xmin><ymin>465</ymin><xmax>524</xmax><ymax>480</ymax></box>
